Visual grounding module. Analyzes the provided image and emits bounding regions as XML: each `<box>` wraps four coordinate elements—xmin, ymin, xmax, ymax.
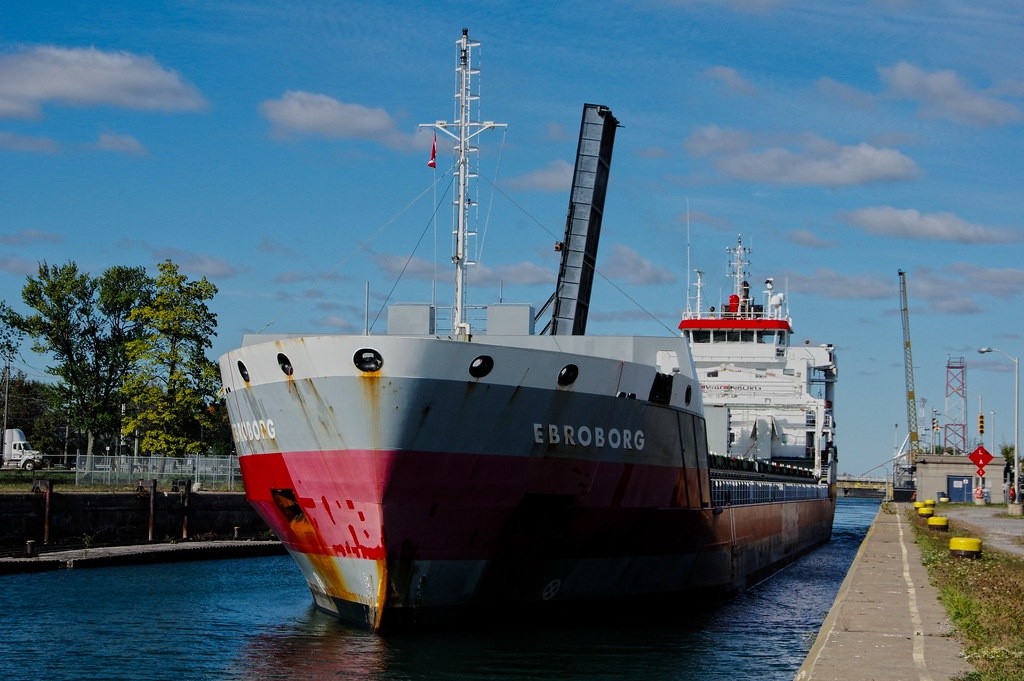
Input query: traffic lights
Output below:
<box><xmin>933</xmin><ymin>418</ymin><xmax>935</xmax><ymax>429</ymax></box>
<box><xmin>937</xmin><ymin>420</ymin><xmax>940</xmax><ymax>431</ymax></box>
<box><xmin>979</xmin><ymin>415</ymin><xmax>985</xmax><ymax>436</ymax></box>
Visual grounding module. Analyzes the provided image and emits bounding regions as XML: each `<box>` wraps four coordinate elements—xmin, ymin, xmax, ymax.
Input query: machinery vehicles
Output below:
<box><xmin>894</xmin><ymin>270</ymin><xmax>920</xmax><ymax>464</ymax></box>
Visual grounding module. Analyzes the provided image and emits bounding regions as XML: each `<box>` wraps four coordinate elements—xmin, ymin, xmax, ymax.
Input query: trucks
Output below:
<box><xmin>0</xmin><ymin>430</ymin><xmax>44</xmax><ymax>470</ymax></box>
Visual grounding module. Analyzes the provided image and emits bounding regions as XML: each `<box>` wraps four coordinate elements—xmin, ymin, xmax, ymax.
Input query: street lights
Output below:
<box><xmin>978</xmin><ymin>347</ymin><xmax>1019</xmax><ymax>501</ymax></box>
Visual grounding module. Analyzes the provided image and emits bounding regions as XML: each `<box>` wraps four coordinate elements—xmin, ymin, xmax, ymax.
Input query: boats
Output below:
<box><xmin>841</xmin><ymin>477</ymin><xmax>881</xmax><ymax>498</ymax></box>
<box><xmin>221</xmin><ymin>29</ymin><xmax>837</xmax><ymax>634</ymax></box>
